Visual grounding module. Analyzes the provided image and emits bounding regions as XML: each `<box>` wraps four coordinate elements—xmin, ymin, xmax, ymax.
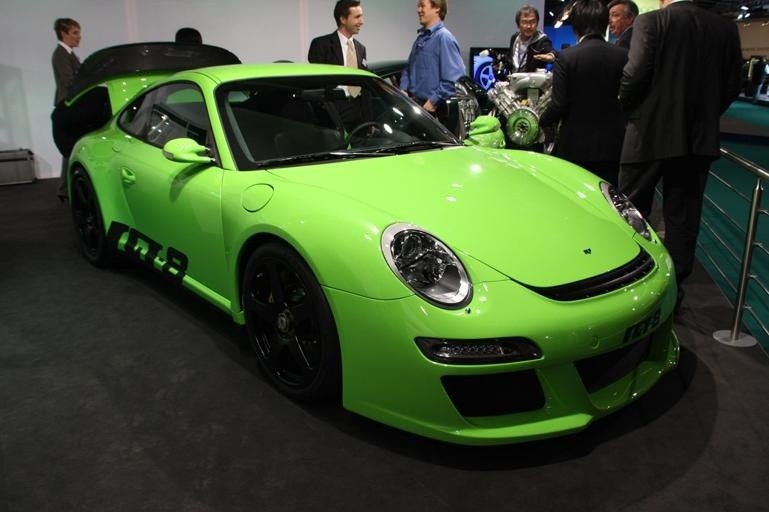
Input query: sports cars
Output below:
<box><xmin>64</xmin><ymin>42</ymin><xmax>682</xmax><ymax>447</ymax></box>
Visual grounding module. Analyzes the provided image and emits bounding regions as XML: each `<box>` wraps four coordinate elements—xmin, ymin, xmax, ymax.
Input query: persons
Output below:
<box><xmin>605</xmin><ymin>0</ymin><xmax>640</xmax><ymax>48</ymax></box>
<box><xmin>395</xmin><ymin>1</ymin><xmax>468</xmax><ymax>137</ymax></box>
<box><xmin>614</xmin><ymin>0</ymin><xmax>747</xmax><ymax>312</ymax></box>
<box><xmin>506</xmin><ymin>6</ymin><xmax>556</xmax><ymax>77</ymax></box>
<box><xmin>48</xmin><ymin>15</ymin><xmax>84</xmax><ymax>200</ymax></box>
<box><xmin>535</xmin><ymin>0</ymin><xmax>628</xmax><ymax>190</ymax></box>
<box><xmin>304</xmin><ymin>2</ymin><xmax>370</xmax><ymax>129</ymax></box>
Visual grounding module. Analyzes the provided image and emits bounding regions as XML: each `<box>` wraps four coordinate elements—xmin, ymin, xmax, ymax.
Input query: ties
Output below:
<box><xmin>345</xmin><ymin>39</ymin><xmax>364</xmax><ymax>97</ymax></box>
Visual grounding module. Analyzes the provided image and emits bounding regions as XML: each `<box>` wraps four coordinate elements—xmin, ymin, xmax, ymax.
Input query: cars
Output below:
<box><xmin>303</xmin><ymin>59</ymin><xmax>498</xmax><ymax>145</ymax></box>
